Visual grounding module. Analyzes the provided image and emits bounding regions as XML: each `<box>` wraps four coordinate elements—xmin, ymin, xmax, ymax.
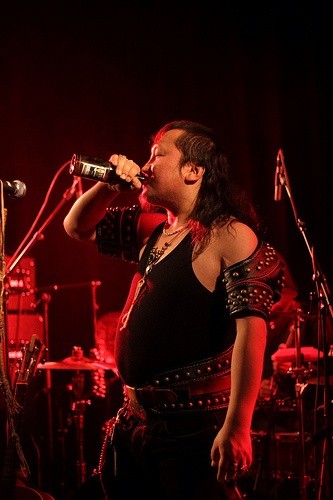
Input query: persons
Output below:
<box><xmin>62</xmin><ymin>121</ymin><xmax>285</xmax><ymax>500</ymax></box>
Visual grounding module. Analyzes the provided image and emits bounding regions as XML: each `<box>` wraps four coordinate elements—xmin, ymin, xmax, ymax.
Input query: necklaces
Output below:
<box><xmin>149</xmin><ymin>219</ymin><xmax>189</xmax><ymax>266</ymax></box>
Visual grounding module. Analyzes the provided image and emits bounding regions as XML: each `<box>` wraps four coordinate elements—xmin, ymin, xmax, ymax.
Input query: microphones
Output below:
<box><xmin>1</xmin><ymin>180</ymin><xmax>27</xmax><ymax>200</ymax></box>
<box><xmin>274</xmin><ymin>150</ymin><xmax>283</xmax><ymax>201</ymax></box>
<box><xmin>73</xmin><ymin>176</ymin><xmax>83</xmax><ymax>199</ymax></box>
